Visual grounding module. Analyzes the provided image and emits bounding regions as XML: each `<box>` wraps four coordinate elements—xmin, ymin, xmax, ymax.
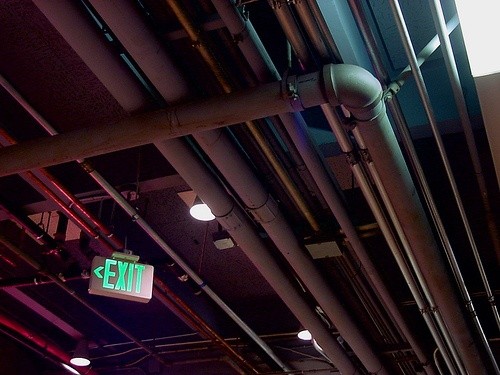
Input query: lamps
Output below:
<box><xmin>190</xmin><ymin>194</ymin><xmax>216</xmax><ymax>221</ymax></box>
<box><xmin>297</xmin><ymin>324</ymin><xmax>312</xmax><ymax>341</ymax></box>
<box><xmin>70</xmin><ymin>335</ymin><xmax>90</xmax><ymax>367</ymax></box>
